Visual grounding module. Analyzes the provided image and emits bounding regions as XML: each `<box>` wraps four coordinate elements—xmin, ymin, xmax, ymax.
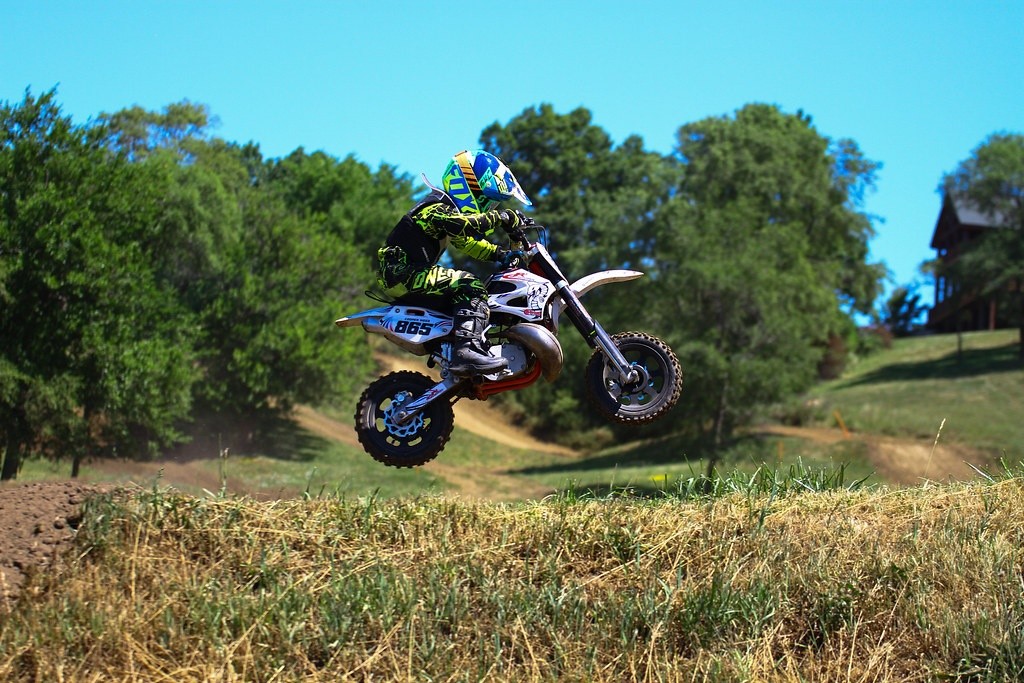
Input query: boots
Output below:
<box><xmin>453</xmin><ymin>308</ymin><xmax>509</xmax><ymax>376</ymax></box>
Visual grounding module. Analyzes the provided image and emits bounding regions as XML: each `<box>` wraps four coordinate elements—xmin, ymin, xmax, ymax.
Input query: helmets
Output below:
<box><xmin>442</xmin><ymin>150</ymin><xmax>532</xmax><ymax>241</ymax></box>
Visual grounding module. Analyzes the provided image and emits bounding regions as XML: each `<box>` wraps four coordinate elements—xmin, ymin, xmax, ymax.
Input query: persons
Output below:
<box><xmin>378</xmin><ymin>150</ymin><xmax>535</xmax><ymax>376</ymax></box>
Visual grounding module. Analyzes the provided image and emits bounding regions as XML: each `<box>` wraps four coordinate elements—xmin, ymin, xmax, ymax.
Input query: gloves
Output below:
<box><xmin>498</xmin><ymin>208</ymin><xmax>520</xmax><ymax>232</ymax></box>
<box><xmin>500</xmin><ymin>249</ymin><xmax>529</xmax><ymax>269</ymax></box>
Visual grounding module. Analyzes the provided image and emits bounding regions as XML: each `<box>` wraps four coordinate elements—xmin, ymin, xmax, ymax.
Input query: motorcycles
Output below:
<box><xmin>335</xmin><ymin>210</ymin><xmax>684</xmax><ymax>469</ymax></box>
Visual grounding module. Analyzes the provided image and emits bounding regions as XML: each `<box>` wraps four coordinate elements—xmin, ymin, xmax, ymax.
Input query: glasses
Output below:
<box><xmin>475</xmin><ymin>194</ymin><xmax>501</xmax><ymax>212</ymax></box>
